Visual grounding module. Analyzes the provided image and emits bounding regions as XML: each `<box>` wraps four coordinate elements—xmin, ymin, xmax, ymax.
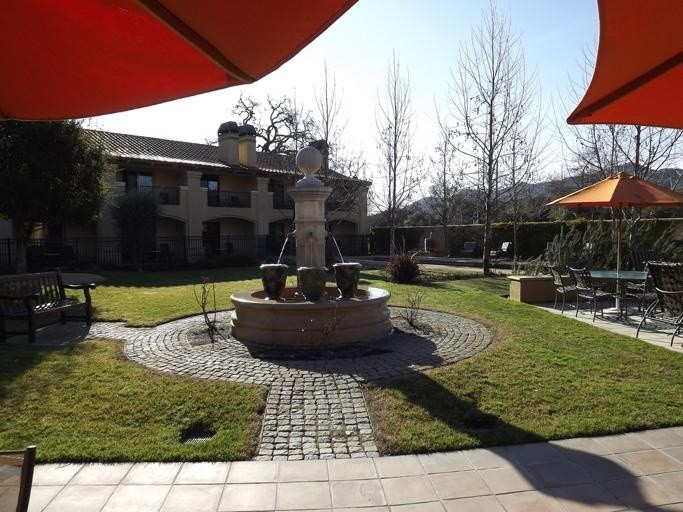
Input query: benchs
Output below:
<box><xmin>489</xmin><ymin>241</ymin><xmax>513</xmax><ymax>259</ymax></box>
<box><xmin>456</xmin><ymin>242</ymin><xmax>481</xmax><ymax>259</ymax></box>
<box><xmin>0</xmin><ymin>267</ymin><xmax>96</xmax><ymax>343</ymax></box>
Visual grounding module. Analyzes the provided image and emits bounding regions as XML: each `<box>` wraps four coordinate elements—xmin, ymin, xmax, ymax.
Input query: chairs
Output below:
<box><xmin>0</xmin><ymin>443</ymin><xmax>37</xmax><ymax>512</ymax></box>
<box><xmin>546</xmin><ymin>261</ymin><xmax>683</xmax><ymax>347</ymax></box>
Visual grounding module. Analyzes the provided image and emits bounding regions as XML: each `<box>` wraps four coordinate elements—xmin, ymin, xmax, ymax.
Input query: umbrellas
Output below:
<box><xmin>563</xmin><ymin>0</ymin><xmax>683</xmax><ymax>134</ymax></box>
<box><xmin>544</xmin><ymin>169</ymin><xmax>682</xmax><ymax>309</ymax></box>
<box><xmin>0</xmin><ymin>1</ymin><xmax>358</xmax><ymax>121</ymax></box>
